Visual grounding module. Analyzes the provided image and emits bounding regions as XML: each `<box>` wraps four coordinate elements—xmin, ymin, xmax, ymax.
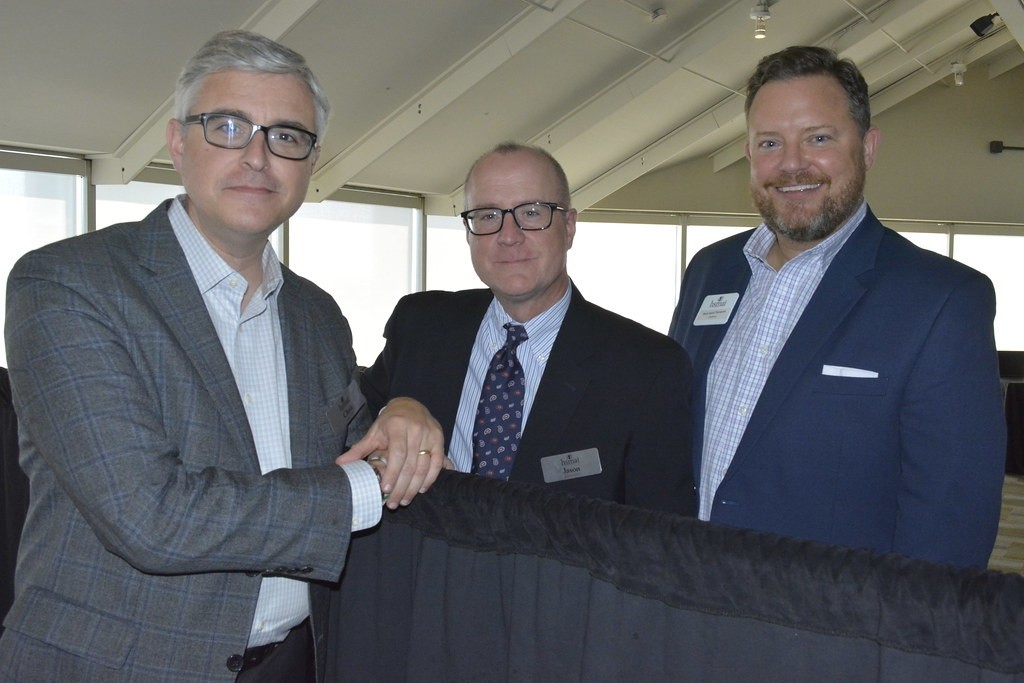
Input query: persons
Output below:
<box><xmin>357</xmin><ymin>144</ymin><xmax>697</xmax><ymax>518</ymax></box>
<box><xmin>0</xmin><ymin>30</ymin><xmax>457</xmax><ymax>682</ymax></box>
<box><xmin>665</xmin><ymin>45</ymin><xmax>1007</xmax><ymax>571</ymax></box>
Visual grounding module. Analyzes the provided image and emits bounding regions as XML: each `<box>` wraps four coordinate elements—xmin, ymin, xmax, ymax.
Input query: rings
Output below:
<box><xmin>419</xmin><ymin>449</ymin><xmax>431</xmax><ymax>456</ymax></box>
<box><xmin>368</xmin><ymin>454</ymin><xmax>389</xmax><ymax>466</ymax></box>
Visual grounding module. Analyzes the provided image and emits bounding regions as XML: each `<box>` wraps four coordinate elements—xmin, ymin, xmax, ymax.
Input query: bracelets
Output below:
<box><xmin>371</xmin><ymin>461</ymin><xmax>383</xmax><ymax>499</ymax></box>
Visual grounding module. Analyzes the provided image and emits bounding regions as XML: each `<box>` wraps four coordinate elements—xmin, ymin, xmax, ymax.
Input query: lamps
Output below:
<box><xmin>748</xmin><ymin>2</ymin><xmax>772</xmax><ymax>40</ymax></box>
<box><xmin>969</xmin><ymin>12</ymin><xmax>999</xmax><ymax>37</ymax></box>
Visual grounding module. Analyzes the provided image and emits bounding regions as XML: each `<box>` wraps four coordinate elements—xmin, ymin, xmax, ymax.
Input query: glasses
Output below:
<box><xmin>460</xmin><ymin>202</ymin><xmax>569</xmax><ymax>236</ymax></box>
<box><xmin>186</xmin><ymin>114</ymin><xmax>317</xmax><ymax>160</ymax></box>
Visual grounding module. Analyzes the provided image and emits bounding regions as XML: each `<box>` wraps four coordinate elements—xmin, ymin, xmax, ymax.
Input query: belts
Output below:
<box><xmin>241</xmin><ymin>641</ymin><xmax>275</xmax><ymax>671</ymax></box>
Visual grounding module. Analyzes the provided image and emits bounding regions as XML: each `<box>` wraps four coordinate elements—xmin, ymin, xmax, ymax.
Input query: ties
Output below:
<box><xmin>471</xmin><ymin>322</ymin><xmax>528</xmax><ymax>481</ymax></box>
